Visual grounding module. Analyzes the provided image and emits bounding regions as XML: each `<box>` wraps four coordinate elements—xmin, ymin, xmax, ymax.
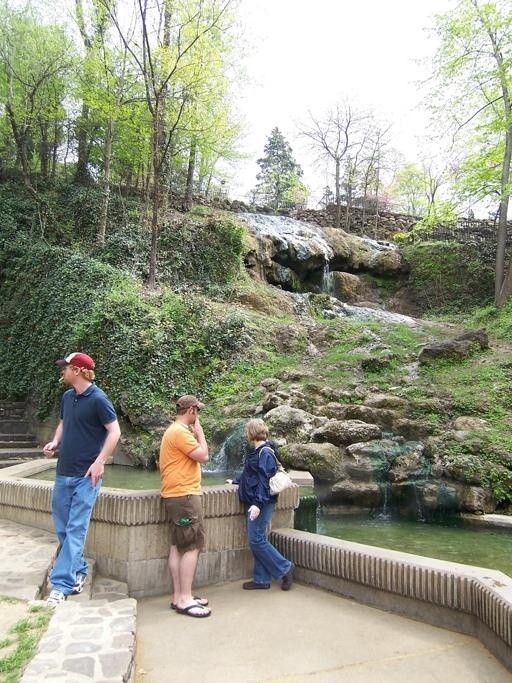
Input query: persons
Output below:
<box><xmin>159</xmin><ymin>394</ymin><xmax>212</xmax><ymax>619</ymax></box>
<box><xmin>41</xmin><ymin>351</ymin><xmax>122</xmax><ymax>604</ymax></box>
<box><xmin>224</xmin><ymin>417</ymin><xmax>296</xmax><ymax>590</ymax></box>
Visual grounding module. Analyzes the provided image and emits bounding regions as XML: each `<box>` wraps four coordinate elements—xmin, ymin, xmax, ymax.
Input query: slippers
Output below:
<box><xmin>171</xmin><ymin>595</ymin><xmax>211</xmax><ymax>617</ymax></box>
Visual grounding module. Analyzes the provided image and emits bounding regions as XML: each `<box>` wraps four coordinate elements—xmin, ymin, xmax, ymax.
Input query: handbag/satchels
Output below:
<box><xmin>269</xmin><ymin>471</ymin><xmax>292</xmax><ymax>495</ymax></box>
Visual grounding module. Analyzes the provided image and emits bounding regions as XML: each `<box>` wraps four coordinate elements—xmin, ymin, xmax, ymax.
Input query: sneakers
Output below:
<box><xmin>46</xmin><ymin>589</ymin><xmax>67</xmax><ymax>603</ymax></box>
<box><xmin>282</xmin><ymin>562</ymin><xmax>295</xmax><ymax>590</ymax></box>
<box><xmin>72</xmin><ymin>573</ymin><xmax>86</xmax><ymax>593</ymax></box>
<box><xmin>244</xmin><ymin>582</ymin><xmax>270</xmax><ymax>589</ymax></box>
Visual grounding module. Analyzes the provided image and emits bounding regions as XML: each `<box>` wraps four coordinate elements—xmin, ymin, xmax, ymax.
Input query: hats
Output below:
<box><xmin>176</xmin><ymin>395</ymin><xmax>206</xmax><ymax>410</ymax></box>
<box><xmin>55</xmin><ymin>353</ymin><xmax>95</xmax><ymax>370</ymax></box>
